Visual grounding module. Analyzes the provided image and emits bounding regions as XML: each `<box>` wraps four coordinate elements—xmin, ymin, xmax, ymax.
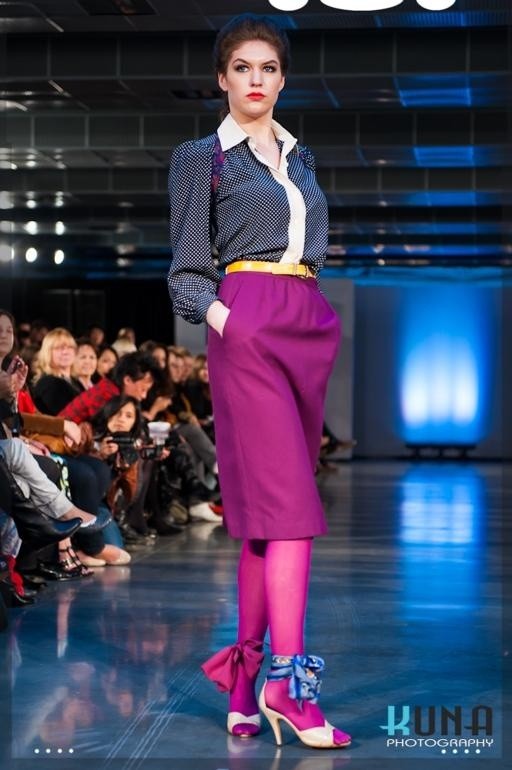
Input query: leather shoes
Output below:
<box><xmin>119</xmin><ymin>511</ymin><xmax>183</xmax><ymax>546</ymax></box>
<box><xmin>189</xmin><ymin>503</ymin><xmax>223</xmax><ymax>523</ymax></box>
<box><xmin>82</xmin><ymin>549</ymin><xmax>131</xmax><ymax>567</ymax></box>
<box><xmin>1</xmin><ymin>547</ymin><xmax>92</xmax><ymax>608</ymax></box>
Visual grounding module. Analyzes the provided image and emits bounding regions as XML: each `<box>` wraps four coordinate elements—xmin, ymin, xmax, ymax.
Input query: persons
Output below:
<box><xmin>2</xmin><ymin>314</ymin><xmax>359</xmax><ymax>610</ymax></box>
<box><xmin>166</xmin><ymin>10</ymin><xmax>357</xmax><ymax>753</ymax></box>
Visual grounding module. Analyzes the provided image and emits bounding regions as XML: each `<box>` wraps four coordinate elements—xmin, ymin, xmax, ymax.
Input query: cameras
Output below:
<box><xmin>108</xmin><ymin>431</ymin><xmax>136</xmax><ymax>447</ymax></box>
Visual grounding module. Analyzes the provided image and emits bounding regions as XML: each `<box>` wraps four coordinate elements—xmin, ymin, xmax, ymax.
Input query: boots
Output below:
<box><xmin>0</xmin><ymin>456</ymin><xmax>83</xmax><ymax>552</ymax></box>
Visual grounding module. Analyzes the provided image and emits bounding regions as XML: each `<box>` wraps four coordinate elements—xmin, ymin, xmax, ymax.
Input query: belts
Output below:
<box><xmin>225</xmin><ymin>260</ymin><xmax>318</xmax><ymax>280</ymax></box>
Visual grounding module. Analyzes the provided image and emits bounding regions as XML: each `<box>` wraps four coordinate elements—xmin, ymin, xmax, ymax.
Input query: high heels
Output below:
<box><xmin>257</xmin><ymin>678</ymin><xmax>353</xmax><ymax>749</ymax></box>
<box><xmin>226</xmin><ymin>711</ymin><xmax>262</xmax><ymax>738</ymax></box>
